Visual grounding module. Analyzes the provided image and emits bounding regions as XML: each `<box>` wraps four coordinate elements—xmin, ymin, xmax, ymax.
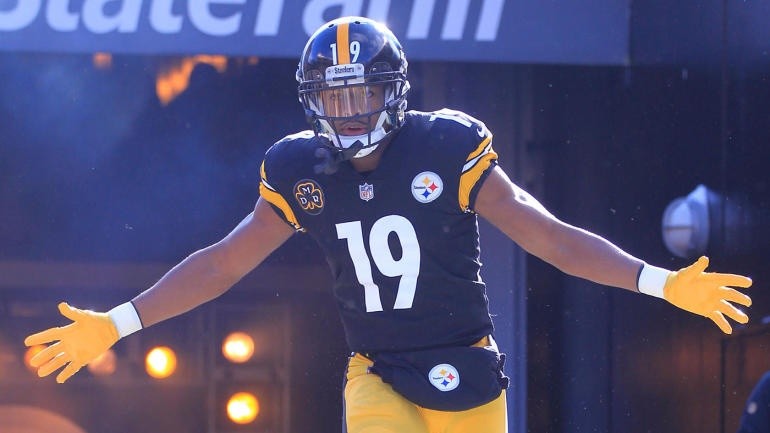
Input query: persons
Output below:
<box><xmin>736</xmin><ymin>317</ymin><xmax>770</xmax><ymax>433</ymax></box>
<box><xmin>22</xmin><ymin>17</ymin><xmax>752</xmax><ymax>433</ymax></box>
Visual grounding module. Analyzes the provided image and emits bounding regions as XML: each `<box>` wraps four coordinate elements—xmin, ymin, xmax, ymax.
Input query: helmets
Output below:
<box><xmin>295</xmin><ymin>15</ymin><xmax>411</xmax><ymax>159</ymax></box>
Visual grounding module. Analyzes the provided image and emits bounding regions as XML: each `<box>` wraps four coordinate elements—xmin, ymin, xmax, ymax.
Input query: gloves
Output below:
<box><xmin>25</xmin><ymin>301</ymin><xmax>144</xmax><ymax>383</ymax></box>
<box><xmin>637</xmin><ymin>256</ymin><xmax>752</xmax><ymax>334</ymax></box>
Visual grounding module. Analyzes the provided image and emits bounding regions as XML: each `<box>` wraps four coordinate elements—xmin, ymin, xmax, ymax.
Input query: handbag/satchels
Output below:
<box><xmin>356</xmin><ymin>330</ymin><xmax>510</xmax><ymax>411</ymax></box>
